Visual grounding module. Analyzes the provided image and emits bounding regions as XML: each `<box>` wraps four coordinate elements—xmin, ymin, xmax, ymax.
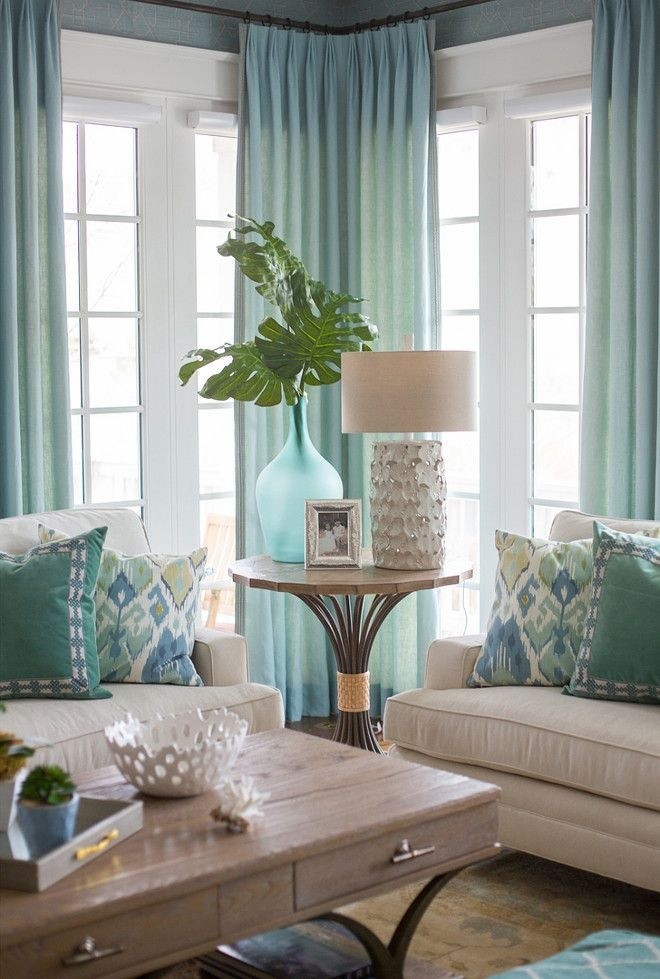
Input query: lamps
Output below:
<box><xmin>341</xmin><ymin>332</ymin><xmax>477</xmax><ymax>570</ymax></box>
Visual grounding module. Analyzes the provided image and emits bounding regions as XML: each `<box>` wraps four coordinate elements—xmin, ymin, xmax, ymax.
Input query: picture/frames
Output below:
<box><xmin>304</xmin><ymin>498</ymin><xmax>362</xmax><ymax>571</ymax></box>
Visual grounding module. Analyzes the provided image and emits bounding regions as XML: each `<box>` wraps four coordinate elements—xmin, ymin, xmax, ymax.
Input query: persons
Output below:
<box><xmin>333</xmin><ymin>519</ymin><xmax>346</xmax><ymax>552</ymax></box>
<box><xmin>320</xmin><ymin>521</ymin><xmax>336</xmax><ymax>554</ymax></box>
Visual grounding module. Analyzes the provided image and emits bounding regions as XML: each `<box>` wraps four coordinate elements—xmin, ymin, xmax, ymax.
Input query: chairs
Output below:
<box><xmin>201</xmin><ymin>511</ymin><xmax>236</xmax><ymax>627</ymax></box>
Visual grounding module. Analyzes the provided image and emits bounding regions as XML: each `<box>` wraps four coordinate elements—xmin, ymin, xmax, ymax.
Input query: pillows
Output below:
<box><xmin>561</xmin><ymin>519</ymin><xmax>660</xmax><ymax>705</ymax></box>
<box><xmin>0</xmin><ymin>526</ymin><xmax>113</xmax><ymax>700</ymax></box>
<box><xmin>37</xmin><ymin>523</ymin><xmax>209</xmax><ymax>688</ymax></box>
<box><xmin>466</xmin><ymin>527</ymin><xmax>655</xmax><ymax>689</ymax></box>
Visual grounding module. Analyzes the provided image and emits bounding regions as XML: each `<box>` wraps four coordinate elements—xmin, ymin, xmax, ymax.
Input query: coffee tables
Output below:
<box><xmin>0</xmin><ymin>723</ymin><xmax>505</xmax><ymax>979</ymax></box>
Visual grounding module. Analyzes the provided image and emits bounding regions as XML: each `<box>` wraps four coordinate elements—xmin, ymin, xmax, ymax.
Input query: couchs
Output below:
<box><xmin>0</xmin><ymin>507</ymin><xmax>284</xmax><ymax>771</ymax></box>
<box><xmin>382</xmin><ymin>509</ymin><xmax>660</xmax><ymax>894</ymax></box>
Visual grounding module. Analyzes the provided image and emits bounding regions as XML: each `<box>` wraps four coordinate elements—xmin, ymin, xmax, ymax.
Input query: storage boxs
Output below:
<box><xmin>0</xmin><ymin>793</ymin><xmax>144</xmax><ymax>892</ymax></box>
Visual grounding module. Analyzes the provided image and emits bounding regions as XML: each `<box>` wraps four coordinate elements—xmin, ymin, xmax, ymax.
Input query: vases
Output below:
<box><xmin>257</xmin><ymin>393</ymin><xmax>343</xmax><ymax>563</ymax></box>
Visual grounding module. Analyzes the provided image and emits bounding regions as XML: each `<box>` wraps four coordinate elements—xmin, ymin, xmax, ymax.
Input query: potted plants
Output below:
<box><xmin>0</xmin><ymin>732</ymin><xmax>54</xmax><ymax>833</ymax></box>
<box><xmin>16</xmin><ymin>763</ymin><xmax>79</xmax><ymax>857</ymax></box>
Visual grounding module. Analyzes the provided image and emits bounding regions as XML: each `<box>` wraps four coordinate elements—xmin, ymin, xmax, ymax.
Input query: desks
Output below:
<box><xmin>227</xmin><ymin>554</ymin><xmax>474</xmax><ymax>756</ymax></box>
